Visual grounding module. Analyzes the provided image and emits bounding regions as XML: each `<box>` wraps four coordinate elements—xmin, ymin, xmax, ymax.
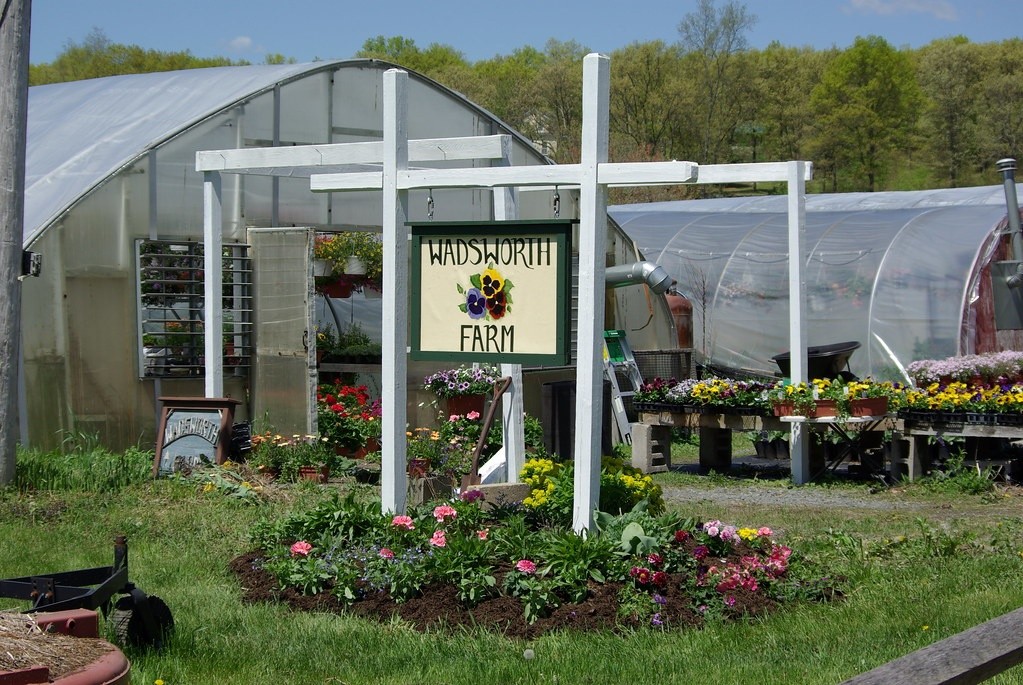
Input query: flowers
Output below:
<box><xmin>408</xmin><ymin>411</ymin><xmax>490</xmax><ymax>471</ymax></box>
<box><xmin>252</xmin><ymin>429</ymin><xmax>340</xmax><ymax>474</ymax></box>
<box><xmin>143</xmin><ymin>244</ymin><xmax>234</xmax><ymax>307</ymax></box>
<box><xmin>316</xmin><ymin>322</ymin><xmax>333</xmax><ymax>347</ymax></box>
<box><xmin>420</xmin><ymin>361</ymin><xmax>503</xmax><ymax>395</ymax></box>
<box><xmin>314</xmin><ymin>228</ymin><xmax>383</xmax><ymax>278</ymax></box>
<box><xmin>633</xmin><ymin>348</ymin><xmax>1023</xmax><ymax>409</ymax></box>
<box><xmin>317</xmin><ymin>374</ymin><xmax>382</xmax><ymax>436</ymax></box>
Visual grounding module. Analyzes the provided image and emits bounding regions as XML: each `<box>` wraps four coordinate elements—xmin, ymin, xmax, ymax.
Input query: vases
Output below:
<box><xmin>363</xmin><ymin>284</ymin><xmax>382</xmax><ymax>299</ymax></box>
<box><xmin>335</xmin><ymin>436</ymin><xmax>348</xmax><ymax>455</ymax></box>
<box><xmin>938</xmin><ymin>375</ymin><xmax>956</xmax><ymax>389</ymax></box>
<box><xmin>316</xmin><ymin>349</ymin><xmax>324</xmax><ymax>369</ymax></box>
<box><xmin>342</xmin><ymin>254</ymin><xmax>369</xmax><ymax>275</ymax></box>
<box><xmin>408</xmin><ymin>457</ymin><xmax>431</xmax><ymax>478</ymax></box>
<box><xmin>914</xmin><ymin>375</ymin><xmax>931</xmax><ymax>388</ymax></box>
<box><xmin>630</xmin><ymin>392</ymin><xmax>1023</xmax><ymax>438</ymax></box>
<box><xmin>968</xmin><ymin>375</ymin><xmax>987</xmax><ymax>389</ymax></box>
<box><xmin>1012</xmin><ymin>370</ymin><xmax>1023</xmax><ymax>382</ymax></box>
<box><xmin>361</xmin><ymin>440</ymin><xmax>379</xmax><ymax>454</ymax></box>
<box><xmin>315</xmin><ymin>257</ymin><xmax>334</xmax><ymax>277</ymax></box>
<box><xmin>445</xmin><ymin>393</ymin><xmax>487</xmax><ymax>421</ymax></box>
<box><xmin>989</xmin><ymin>374</ymin><xmax>1010</xmax><ymax>387</ymax></box>
<box><xmin>329</xmin><ymin>288</ymin><xmax>353</xmax><ymax>298</ymax></box>
<box><xmin>300</xmin><ymin>463</ymin><xmax>333</xmax><ymax>483</ymax></box>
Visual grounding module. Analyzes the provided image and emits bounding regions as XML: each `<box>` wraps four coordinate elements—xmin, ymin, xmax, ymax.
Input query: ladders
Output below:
<box><xmin>602</xmin><ymin>329</ymin><xmax>646</xmax><ymax>446</ymax></box>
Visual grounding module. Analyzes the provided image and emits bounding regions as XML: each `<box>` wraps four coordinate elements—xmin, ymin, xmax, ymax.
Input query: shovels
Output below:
<box><xmin>461</xmin><ymin>376</ymin><xmax>512</xmax><ymax>497</ymax></box>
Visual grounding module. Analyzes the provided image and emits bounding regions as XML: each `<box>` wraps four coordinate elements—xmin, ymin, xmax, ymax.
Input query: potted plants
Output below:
<box><xmin>196</xmin><ymin>313</ymin><xmax>235</xmax><ymax>364</ymax></box>
